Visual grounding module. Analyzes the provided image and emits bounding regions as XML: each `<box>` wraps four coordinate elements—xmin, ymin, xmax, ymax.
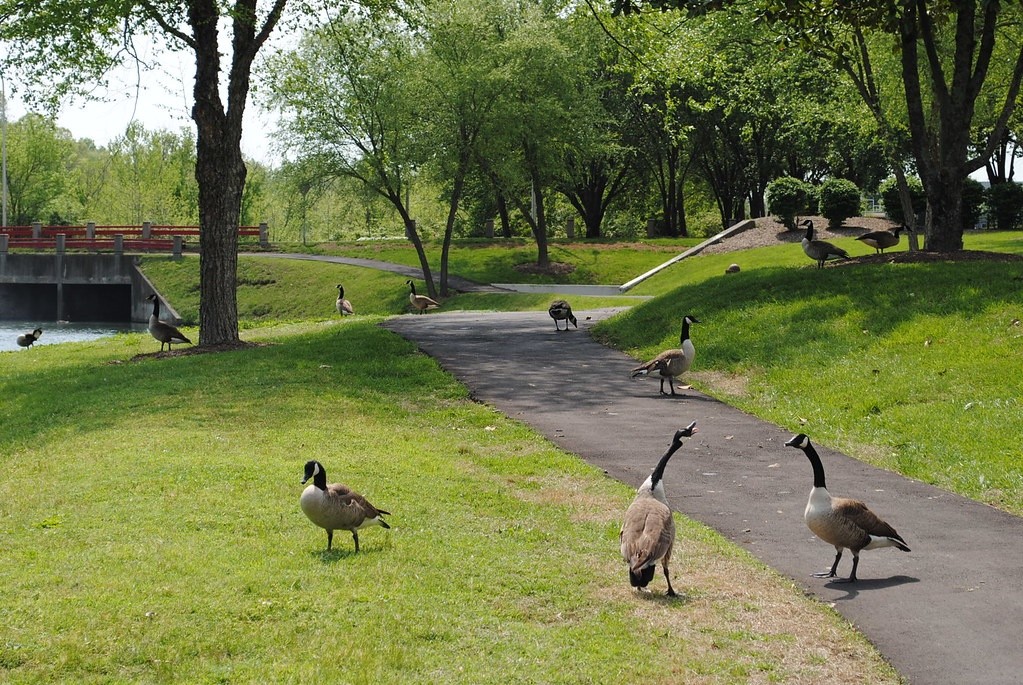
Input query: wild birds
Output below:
<box><xmin>784</xmin><ymin>432</ymin><xmax>910</xmax><ymax>583</ymax></box>
<box><xmin>300</xmin><ymin>460</ymin><xmax>391</xmax><ymax>551</ymax></box>
<box><xmin>632</xmin><ymin>315</ymin><xmax>702</xmax><ymax>395</ymax></box>
<box><xmin>798</xmin><ymin>219</ymin><xmax>851</xmax><ymax>268</ymax></box>
<box><xmin>404</xmin><ymin>280</ymin><xmax>444</xmax><ymax>314</ymax></box>
<box><xmin>854</xmin><ymin>226</ymin><xmax>913</xmax><ymax>253</ymax></box>
<box><xmin>549</xmin><ymin>300</ymin><xmax>577</xmax><ymax>330</ymax></box>
<box><xmin>617</xmin><ymin>418</ymin><xmax>701</xmax><ymax>596</ymax></box>
<box><xmin>145</xmin><ymin>294</ymin><xmax>193</xmax><ymax>351</ymax></box>
<box><xmin>335</xmin><ymin>284</ymin><xmax>355</xmax><ymax>318</ymax></box>
<box><xmin>16</xmin><ymin>327</ymin><xmax>42</xmax><ymax>350</ymax></box>
